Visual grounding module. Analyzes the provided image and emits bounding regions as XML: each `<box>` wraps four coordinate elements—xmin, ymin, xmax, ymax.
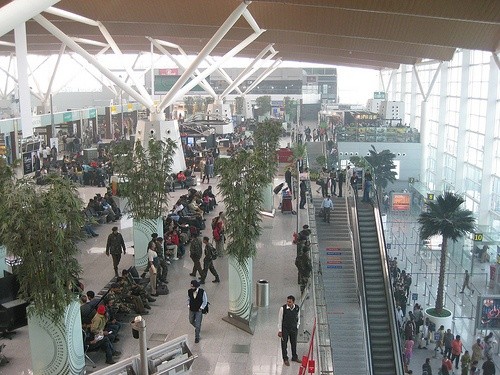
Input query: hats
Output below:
<box><xmin>97</xmin><ymin>305</ymin><xmax>105</xmax><ymax>314</ymax></box>
<box><xmin>303</xmin><ymin>246</ymin><xmax>310</xmax><ymax>252</ymax></box>
<box><xmin>111</xmin><ymin>283</ymin><xmax>118</xmax><ymax>289</ymax></box>
<box><xmin>300</xmin><ymin>235</ymin><xmax>307</xmax><ymax>240</ymax></box>
<box><xmin>303</xmin><ymin>224</ymin><xmax>309</xmax><ymax>229</ymax></box>
<box><xmin>90</xmin><ymin>193</ymin><xmax>103</xmax><ymax>202</ymax></box>
<box><xmin>151</xmin><ymin>233</ymin><xmax>163</xmax><ymax>242</ymax></box>
<box><xmin>112</xmin><ymin>227</ymin><xmax>118</xmax><ymax>231</ymax></box>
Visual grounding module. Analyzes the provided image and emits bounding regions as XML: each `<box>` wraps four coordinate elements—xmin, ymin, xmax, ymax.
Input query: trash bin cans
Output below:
<box><xmin>256</xmin><ymin>279</ymin><xmax>271</xmax><ymax>307</ymax></box>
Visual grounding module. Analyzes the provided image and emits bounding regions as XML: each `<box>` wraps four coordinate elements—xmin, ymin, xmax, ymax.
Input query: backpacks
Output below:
<box><xmin>213</xmin><ymin>227</ymin><xmax>224</xmax><ymax>241</ymax></box>
<box><xmin>206</xmin><ymin>244</ymin><xmax>218</xmax><ymax>260</ymax></box>
<box><xmin>294</xmin><ymin>253</ymin><xmax>310</xmax><ymax>277</ymax></box>
<box><xmin>211</xmin><ymin>216</ymin><xmax>219</xmax><ymax>229</ymax></box>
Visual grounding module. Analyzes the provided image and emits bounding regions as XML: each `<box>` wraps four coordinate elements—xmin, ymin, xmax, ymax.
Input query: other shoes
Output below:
<box><xmin>159</xmin><ymin>254</ymin><xmax>183</xmax><ymax>283</ymax></box>
<box><xmin>140</xmin><ymin>290</ymin><xmax>160</xmax><ymax>315</ymax></box>
<box><xmin>291</xmin><ymin>358</ymin><xmax>301</xmax><ymax>363</ymax></box>
<box><xmin>199</xmin><ymin>279</ymin><xmax>219</xmax><ymax>284</ymax></box>
<box><xmin>104</xmin><ymin>332</ymin><xmax>121</xmax><ymax>364</ymax></box>
<box><xmin>92</xmin><ymin>232</ymin><xmax>98</xmax><ymax>237</ymax></box>
<box><xmin>190</xmin><ymin>272</ymin><xmax>203</xmax><ymax>280</ymax></box>
<box><xmin>195</xmin><ymin>337</ymin><xmax>199</xmax><ymax>343</ymax></box>
<box><xmin>284</xmin><ymin>360</ymin><xmax>290</xmax><ymax>366</ymax></box>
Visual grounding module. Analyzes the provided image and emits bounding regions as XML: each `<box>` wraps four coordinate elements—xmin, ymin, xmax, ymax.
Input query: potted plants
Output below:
<box><xmin>285</xmin><ymin>97</ymin><xmax>298</xmax><ymax>135</ymax></box>
<box><xmin>106</xmin><ymin>136</ymin><xmax>177</xmax><ymax>274</ymax></box>
<box><xmin>365</xmin><ymin>145</ymin><xmax>399</xmax><ymax>221</ymax></box>
<box><xmin>320</xmin><ymin>122</ymin><xmax>329</xmax><ymax>135</ymax></box>
<box><xmin>417</xmin><ymin>183</ymin><xmax>477</xmax><ymax>333</ymax></box>
<box><xmin>0</xmin><ymin>147</ymin><xmax>86</xmax><ymax>375</ymax></box>
<box><xmin>247</xmin><ymin>117</ymin><xmax>284</xmax><ymax>214</ymax></box>
<box><xmin>213</xmin><ymin>147</ymin><xmax>266</xmax><ymax>336</ymax></box>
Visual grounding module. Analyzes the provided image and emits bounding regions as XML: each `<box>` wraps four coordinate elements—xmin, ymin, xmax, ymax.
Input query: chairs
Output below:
<box><xmin>0</xmin><ymin>310</ymin><xmax>17</xmax><ymax>340</ymax></box>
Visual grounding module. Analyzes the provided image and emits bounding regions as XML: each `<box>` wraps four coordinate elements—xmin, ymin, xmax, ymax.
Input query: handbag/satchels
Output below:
<box><xmin>156</xmin><ymin>285</ymin><xmax>169</xmax><ymax>295</ymax></box>
<box><xmin>202</xmin><ymin>302</ymin><xmax>210</xmax><ymax>314</ymax></box>
<box><xmin>319</xmin><ymin>210</ymin><xmax>325</xmax><ymax>218</ymax></box>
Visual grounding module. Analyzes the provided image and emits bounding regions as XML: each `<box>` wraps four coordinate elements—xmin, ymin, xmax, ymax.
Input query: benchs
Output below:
<box><xmin>82</xmin><ymin>207</ymin><xmax>107</xmax><ymax>237</ymax></box>
<box><xmin>81</xmin><ymin>265</ymin><xmax>150</xmax><ymax>369</ymax></box>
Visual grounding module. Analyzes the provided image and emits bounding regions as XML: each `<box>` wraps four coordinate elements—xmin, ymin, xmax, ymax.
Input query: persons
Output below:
<box><xmin>285</xmin><ymin>168</ymin><xmax>306</xmax><ymax>208</ymax></box>
<box><xmin>164</xmin><ymin>146</ymin><xmax>220</xmax><ymax>192</ymax></box>
<box><xmin>460</xmin><ymin>270</ymin><xmax>474</xmax><ymax>294</ymax></box>
<box><xmin>225</xmin><ymin>120</ymin><xmax>257</xmax><ymax>156</ymax></box>
<box><xmin>388</xmin><ymin>255</ymin><xmax>412</xmax><ymax>317</ymax></box>
<box><xmin>80</xmin><ymin>268</ymin><xmax>156</xmax><ymax>364</ymax></box>
<box><xmin>79</xmin><ymin>193</ymin><xmax>122</xmax><ymax>237</ymax></box>
<box><xmin>106</xmin><ymin>227</ymin><xmax>126</xmax><ymax>276</ymax></box>
<box><xmin>44</xmin><ymin>126</ymin><xmax>129</xmax><ymax>186</ymax></box>
<box><xmin>33</xmin><ymin>151</ymin><xmax>39</xmax><ymax>171</ymax></box>
<box><xmin>141</xmin><ymin>186</ymin><xmax>226</xmax><ymax>284</ymax></box>
<box><xmin>305</xmin><ymin>126</ymin><xmax>323</xmax><ymax>142</ymax></box>
<box><xmin>278</xmin><ymin>295</ymin><xmax>302</xmax><ymax>366</ymax></box>
<box><xmin>295</xmin><ymin>225</ymin><xmax>312</xmax><ymax>299</ymax></box>
<box><xmin>461</xmin><ymin>332</ymin><xmax>495</xmax><ymax>375</ymax></box>
<box><xmin>396</xmin><ymin>303</ymin><xmax>462</xmax><ymax>375</ymax></box>
<box><xmin>317</xmin><ymin>167</ymin><xmax>343</xmax><ymax>222</ymax></box>
<box><xmin>186</xmin><ymin>280</ymin><xmax>207</xmax><ymax>343</ymax></box>
<box><xmin>347</xmin><ymin>167</ymin><xmax>372</xmax><ymax>202</ymax></box>
